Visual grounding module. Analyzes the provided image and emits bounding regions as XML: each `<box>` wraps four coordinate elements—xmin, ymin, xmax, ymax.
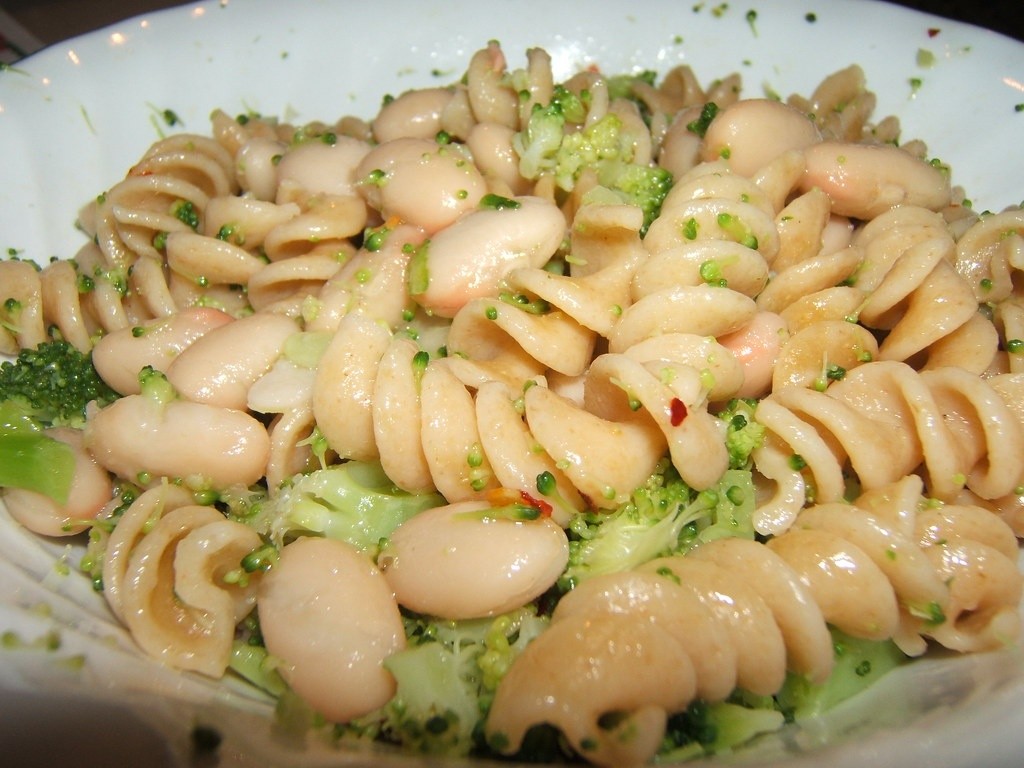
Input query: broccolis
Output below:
<box><xmin>0</xmin><ymin>69</ymin><xmax>916</xmax><ymax>768</ymax></box>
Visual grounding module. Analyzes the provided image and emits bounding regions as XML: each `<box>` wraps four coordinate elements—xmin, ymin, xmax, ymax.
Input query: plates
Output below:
<box><xmin>0</xmin><ymin>0</ymin><xmax>1024</xmax><ymax>768</ymax></box>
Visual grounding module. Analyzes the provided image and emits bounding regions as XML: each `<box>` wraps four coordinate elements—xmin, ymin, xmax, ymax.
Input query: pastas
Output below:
<box><xmin>0</xmin><ymin>40</ymin><xmax>1024</xmax><ymax>768</ymax></box>
